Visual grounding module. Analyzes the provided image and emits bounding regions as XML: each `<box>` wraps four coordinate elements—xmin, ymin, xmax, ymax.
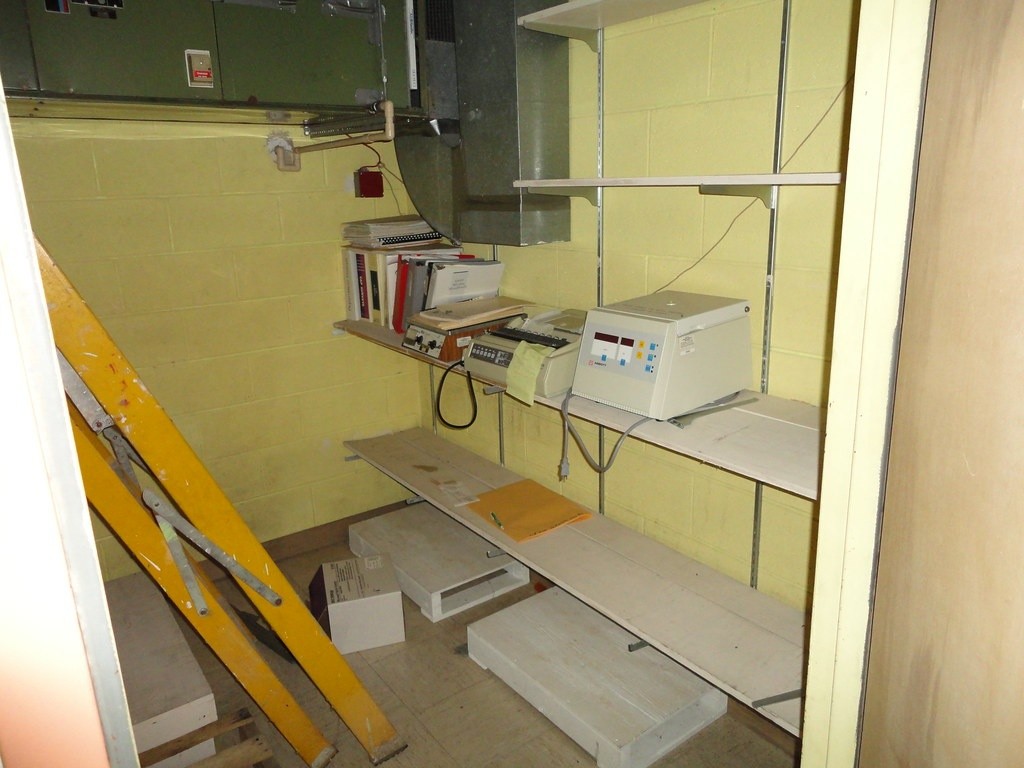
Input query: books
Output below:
<box><xmin>338</xmin><ymin>213</ymin><xmax>504</xmax><ymax>333</ymax></box>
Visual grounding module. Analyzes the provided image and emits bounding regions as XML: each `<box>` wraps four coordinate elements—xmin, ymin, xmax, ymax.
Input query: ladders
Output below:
<box><xmin>32</xmin><ymin>232</ymin><xmax>408</xmax><ymax>768</ymax></box>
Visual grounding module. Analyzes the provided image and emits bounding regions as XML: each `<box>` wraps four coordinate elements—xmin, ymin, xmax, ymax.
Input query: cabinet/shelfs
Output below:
<box><xmin>333</xmin><ymin>1</ymin><xmax>861</xmax><ymax>768</ymax></box>
<box><xmin>0</xmin><ymin>0</ymin><xmax>421</xmax><ymax>112</ymax></box>
